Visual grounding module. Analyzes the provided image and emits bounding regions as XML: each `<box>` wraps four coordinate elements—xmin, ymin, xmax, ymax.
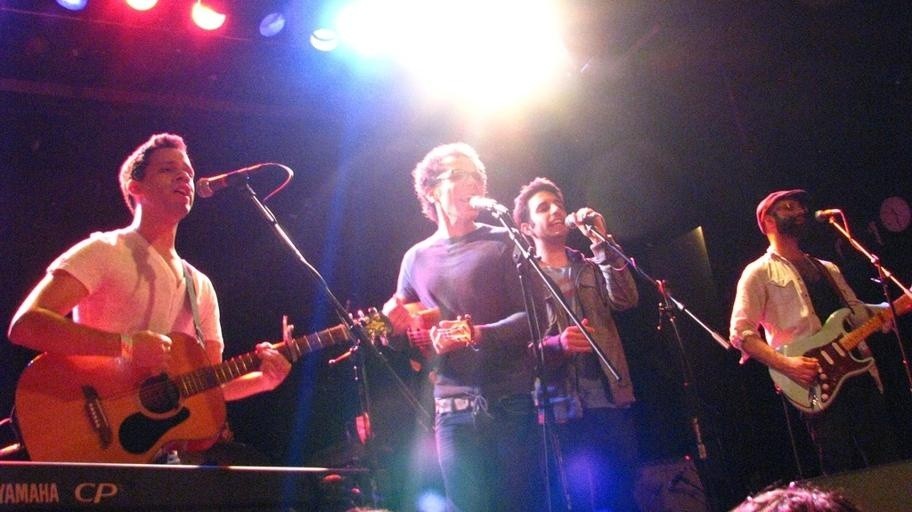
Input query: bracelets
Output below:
<box><xmin>117</xmin><ymin>332</ymin><xmax>134</xmax><ymax>372</ymax></box>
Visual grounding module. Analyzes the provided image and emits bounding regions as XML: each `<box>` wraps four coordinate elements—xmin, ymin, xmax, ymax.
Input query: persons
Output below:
<box><xmin>512</xmin><ymin>175</ymin><xmax>639</xmax><ymax>512</ymax></box>
<box><xmin>729</xmin><ymin>189</ymin><xmax>901</xmax><ymax>478</ymax></box>
<box><xmin>379</xmin><ymin>139</ymin><xmax>550</xmax><ymax>512</ymax></box>
<box><xmin>726</xmin><ymin>480</ymin><xmax>858</xmax><ymax>512</ymax></box>
<box><xmin>7</xmin><ymin>132</ymin><xmax>291</xmax><ymax>466</ymax></box>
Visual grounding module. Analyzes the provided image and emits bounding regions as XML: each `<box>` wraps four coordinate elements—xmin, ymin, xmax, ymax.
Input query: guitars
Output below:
<box><xmin>767</xmin><ymin>288</ymin><xmax>911</xmax><ymax>412</ymax></box>
<box><xmin>17</xmin><ymin>308</ymin><xmax>395</xmax><ymax>463</ymax></box>
<box><xmin>387</xmin><ymin>300</ymin><xmax>474</xmax><ymax>375</ymax></box>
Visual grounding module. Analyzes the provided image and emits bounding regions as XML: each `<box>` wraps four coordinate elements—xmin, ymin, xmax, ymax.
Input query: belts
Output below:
<box><xmin>434</xmin><ymin>395</ymin><xmax>511</xmax><ymax>415</ymax></box>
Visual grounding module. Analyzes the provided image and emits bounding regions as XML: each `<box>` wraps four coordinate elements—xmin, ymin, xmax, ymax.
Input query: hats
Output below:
<box><xmin>756</xmin><ymin>189</ymin><xmax>815</xmax><ymax>236</ymax></box>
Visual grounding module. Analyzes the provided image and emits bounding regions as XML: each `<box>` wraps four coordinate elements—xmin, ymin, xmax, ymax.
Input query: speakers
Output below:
<box><xmin>652</xmin><ymin>225</ymin><xmax>734</xmax><ymax>350</ymax></box>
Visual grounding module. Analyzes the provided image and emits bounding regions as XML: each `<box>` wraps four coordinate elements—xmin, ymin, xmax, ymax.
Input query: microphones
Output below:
<box><xmin>468</xmin><ymin>194</ymin><xmax>511</xmax><ymax>217</ymax></box>
<box><xmin>813</xmin><ymin>207</ymin><xmax>837</xmax><ymax>224</ymax></box>
<box><xmin>193</xmin><ymin>163</ymin><xmax>268</xmax><ymax>198</ymax></box>
<box><xmin>565</xmin><ymin>212</ymin><xmax>600</xmax><ymax>231</ymax></box>
